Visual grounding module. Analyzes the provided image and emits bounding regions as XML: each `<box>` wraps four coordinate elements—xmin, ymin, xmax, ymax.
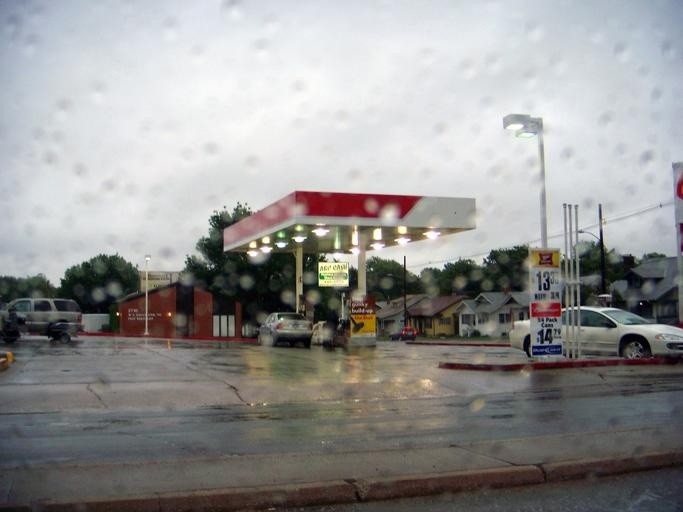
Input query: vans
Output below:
<box><xmin>6</xmin><ymin>298</ymin><xmax>84</xmax><ymax>341</ymax></box>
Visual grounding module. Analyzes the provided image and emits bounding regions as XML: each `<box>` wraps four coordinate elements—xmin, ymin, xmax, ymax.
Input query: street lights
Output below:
<box><xmin>504</xmin><ymin>113</ymin><xmax>548</xmax><ymax>253</ymax></box>
<box><xmin>143</xmin><ymin>255</ymin><xmax>152</xmax><ymax>337</ymax></box>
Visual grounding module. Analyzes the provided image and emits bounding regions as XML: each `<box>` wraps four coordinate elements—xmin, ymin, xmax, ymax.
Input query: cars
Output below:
<box><xmin>511</xmin><ymin>303</ymin><xmax>683</xmax><ymax>362</ymax></box>
<box><xmin>389</xmin><ymin>326</ymin><xmax>416</xmax><ymax>342</ymax></box>
<box><xmin>257</xmin><ymin>311</ymin><xmax>313</xmax><ymax>346</ymax></box>
